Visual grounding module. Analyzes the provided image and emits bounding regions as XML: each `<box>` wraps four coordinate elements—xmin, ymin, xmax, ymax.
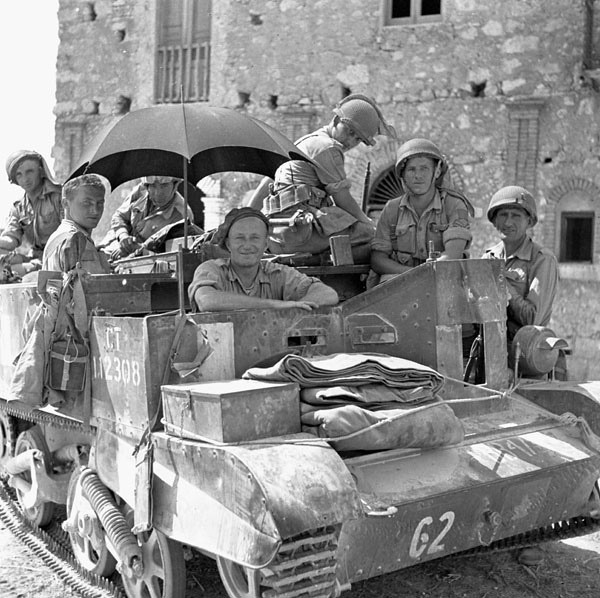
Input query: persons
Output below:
<box><xmin>188</xmin><ymin>206</ymin><xmax>340</xmax><ymax>312</ymax></box>
<box><xmin>0</xmin><ymin>149</ymin><xmax>62</xmax><ymax>284</ymax></box>
<box><xmin>469</xmin><ymin>184</ymin><xmax>558</xmax><ymax>384</ymax></box>
<box><xmin>260</xmin><ymin>97</ymin><xmax>382</xmax><ymax>261</ymax></box>
<box><xmin>367</xmin><ymin>137</ymin><xmax>472</xmax><ymax>291</ymax></box>
<box><xmin>96</xmin><ymin>174</ymin><xmax>196</xmax><ymax>260</ymax></box>
<box><xmin>42</xmin><ymin>175</ymin><xmax>114</xmax><ymax>274</ymax></box>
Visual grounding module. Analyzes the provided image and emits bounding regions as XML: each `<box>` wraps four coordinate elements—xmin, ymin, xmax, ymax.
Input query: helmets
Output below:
<box><xmin>332</xmin><ymin>99</ymin><xmax>380</xmax><ymax>147</ymax></box>
<box><xmin>486</xmin><ymin>185</ymin><xmax>538</xmax><ymax>230</ymax></box>
<box><xmin>140</xmin><ymin>176</ymin><xmax>183</xmax><ymax>187</ymax></box>
<box><xmin>394</xmin><ymin>137</ymin><xmax>448</xmax><ymax>180</ymax></box>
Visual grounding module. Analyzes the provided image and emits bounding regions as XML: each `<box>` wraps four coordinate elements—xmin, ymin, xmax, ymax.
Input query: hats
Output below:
<box><xmin>5</xmin><ymin>149</ymin><xmax>42</xmax><ymax>185</ymax></box>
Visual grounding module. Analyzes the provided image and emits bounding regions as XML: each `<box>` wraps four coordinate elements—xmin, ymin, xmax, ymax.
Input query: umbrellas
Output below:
<box><xmin>62</xmin><ymin>85</ymin><xmax>321</xmax><ymax>251</ymax></box>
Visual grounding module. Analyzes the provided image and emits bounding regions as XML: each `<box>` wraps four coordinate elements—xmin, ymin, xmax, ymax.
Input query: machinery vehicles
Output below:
<box><xmin>0</xmin><ymin>230</ymin><xmax>600</xmax><ymax>596</ymax></box>
